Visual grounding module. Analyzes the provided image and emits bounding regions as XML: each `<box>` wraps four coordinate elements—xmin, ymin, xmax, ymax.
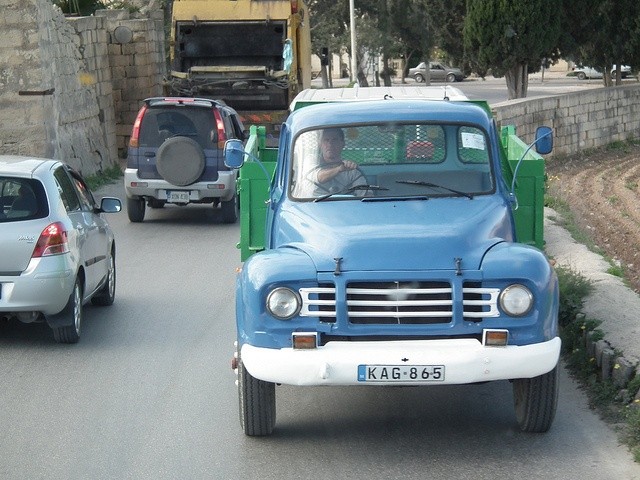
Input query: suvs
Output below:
<box><xmin>124</xmin><ymin>97</ymin><xmax>250</xmax><ymax>223</ymax></box>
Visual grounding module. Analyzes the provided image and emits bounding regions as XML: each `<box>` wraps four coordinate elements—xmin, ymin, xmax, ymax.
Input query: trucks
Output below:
<box><xmin>222</xmin><ymin>95</ymin><xmax>562</xmax><ymax>436</ymax></box>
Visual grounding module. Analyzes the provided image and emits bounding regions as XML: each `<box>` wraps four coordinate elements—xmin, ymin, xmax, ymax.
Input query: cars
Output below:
<box><xmin>572</xmin><ymin>62</ymin><xmax>631</xmax><ymax>80</ymax></box>
<box><xmin>408</xmin><ymin>62</ymin><xmax>465</xmax><ymax>82</ymax></box>
<box><xmin>0</xmin><ymin>155</ymin><xmax>122</xmax><ymax>344</ymax></box>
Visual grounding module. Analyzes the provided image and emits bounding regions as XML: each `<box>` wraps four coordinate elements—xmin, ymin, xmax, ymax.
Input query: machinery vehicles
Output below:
<box><xmin>163</xmin><ymin>0</ymin><xmax>311</xmax><ymax>147</ymax></box>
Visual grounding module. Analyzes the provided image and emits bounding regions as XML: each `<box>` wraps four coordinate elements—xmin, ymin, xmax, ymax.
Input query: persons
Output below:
<box><xmin>300</xmin><ymin>127</ymin><xmax>373</xmax><ymax>196</ymax></box>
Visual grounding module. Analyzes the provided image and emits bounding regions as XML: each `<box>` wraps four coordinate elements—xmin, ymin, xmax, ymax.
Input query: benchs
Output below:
<box><xmin>376</xmin><ymin>172</ymin><xmax>483</xmax><ymax>197</ymax></box>
